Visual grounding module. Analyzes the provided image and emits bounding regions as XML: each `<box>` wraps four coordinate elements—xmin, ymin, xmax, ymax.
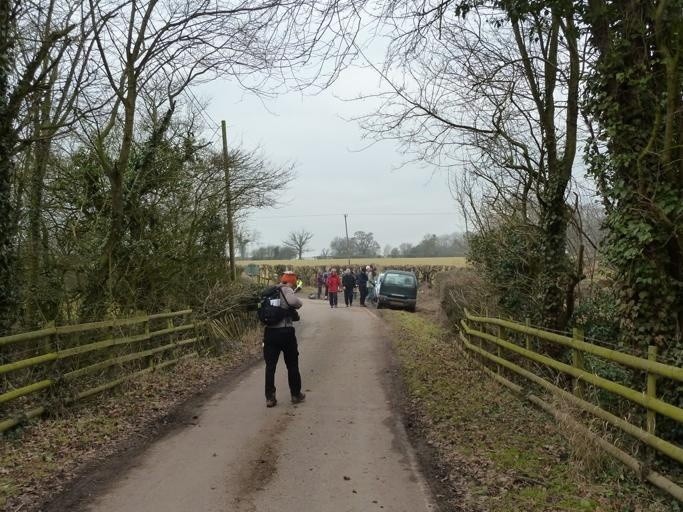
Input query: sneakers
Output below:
<box><xmin>265</xmin><ymin>397</ymin><xmax>278</xmax><ymax>407</ymax></box>
<box><xmin>290</xmin><ymin>390</ymin><xmax>306</xmax><ymax>404</ymax></box>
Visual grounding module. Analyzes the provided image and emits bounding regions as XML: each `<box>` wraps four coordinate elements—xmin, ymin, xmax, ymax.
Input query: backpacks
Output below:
<box><xmin>257</xmin><ymin>285</ymin><xmax>284</xmax><ymax>326</ymax></box>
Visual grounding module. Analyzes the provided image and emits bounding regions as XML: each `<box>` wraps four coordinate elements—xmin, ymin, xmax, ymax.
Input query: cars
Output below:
<box><xmin>373</xmin><ymin>270</ymin><xmax>418</xmax><ymax>312</ymax></box>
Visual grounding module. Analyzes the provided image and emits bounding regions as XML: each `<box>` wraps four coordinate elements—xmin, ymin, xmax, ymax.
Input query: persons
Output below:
<box><xmin>316</xmin><ymin>265</ymin><xmax>377</xmax><ymax>309</ymax></box>
<box><xmin>293</xmin><ymin>277</ymin><xmax>303</xmax><ymax>293</ymax></box>
<box><xmin>257</xmin><ymin>271</ymin><xmax>306</xmax><ymax>407</ymax></box>
